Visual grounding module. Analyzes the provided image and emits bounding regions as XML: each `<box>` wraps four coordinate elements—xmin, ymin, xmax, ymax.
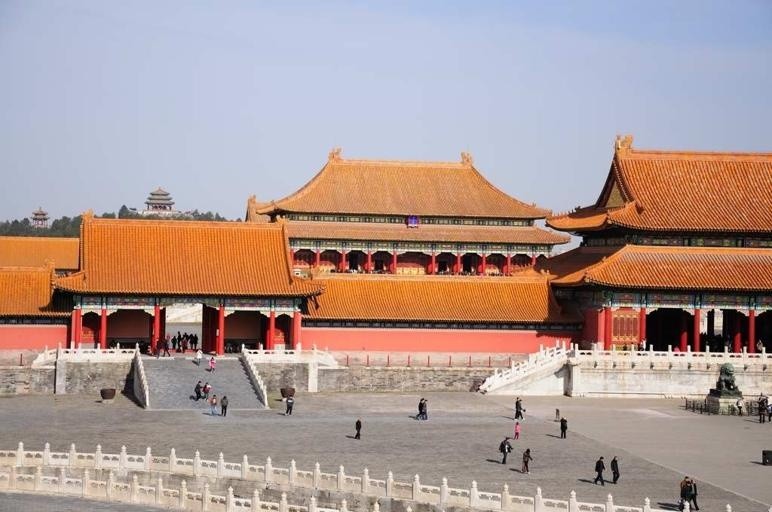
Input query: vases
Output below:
<box><xmin>101</xmin><ymin>388</ymin><xmax>116</xmax><ymax>399</ymax></box>
<box><xmin>280</xmin><ymin>387</ymin><xmax>295</xmax><ymax>398</ymax></box>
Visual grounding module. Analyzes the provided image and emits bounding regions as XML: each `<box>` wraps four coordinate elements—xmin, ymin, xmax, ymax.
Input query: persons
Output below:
<box><xmin>737</xmin><ymin>398</ymin><xmax>744</xmax><ymax>416</ymax></box>
<box><xmin>416</xmin><ymin>398</ymin><xmax>428</xmax><ymax>420</ymax></box>
<box><xmin>523</xmin><ymin>449</ymin><xmax>533</xmax><ymax>474</ymax></box>
<box><xmin>209</xmin><ymin>357</ymin><xmax>216</xmax><ymax>373</ymax></box>
<box><xmin>757</xmin><ymin>398</ymin><xmax>772</xmax><ymax>423</ymax></box>
<box><xmin>514</xmin><ymin>398</ymin><xmax>526</xmax><ymax>420</ymax></box>
<box><xmin>285</xmin><ymin>395</ymin><xmax>294</xmax><ymax>416</ymax></box>
<box><xmin>560</xmin><ymin>416</ymin><xmax>568</xmax><ymax>439</ymax></box>
<box><xmin>679</xmin><ymin>476</ymin><xmax>699</xmax><ymax>511</ymax></box>
<box><xmin>163</xmin><ymin>332</ymin><xmax>198</xmax><ymax>357</ymax></box>
<box><xmin>355</xmin><ymin>419</ymin><xmax>361</xmax><ymax>440</ymax></box>
<box><xmin>756</xmin><ymin>338</ymin><xmax>763</xmax><ymax>353</ymax></box>
<box><xmin>197</xmin><ymin>348</ymin><xmax>203</xmax><ymax>366</ymax></box>
<box><xmin>195</xmin><ymin>381</ymin><xmax>228</xmax><ymax>417</ymax></box>
<box><xmin>514</xmin><ymin>422</ymin><xmax>520</xmax><ymax>440</ymax></box>
<box><xmin>595</xmin><ymin>457</ymin><xmax>605</xmax><ymax>486</ymax></box>
<box><xmin>499</xmin><ymin>437</ymin><xmax>514</xmax><ymax>464</ymax></box>
<box><xmin>611</xmin><ymin>456</ymin><xmax>620</xmax><ymax>484</ymax></box>
